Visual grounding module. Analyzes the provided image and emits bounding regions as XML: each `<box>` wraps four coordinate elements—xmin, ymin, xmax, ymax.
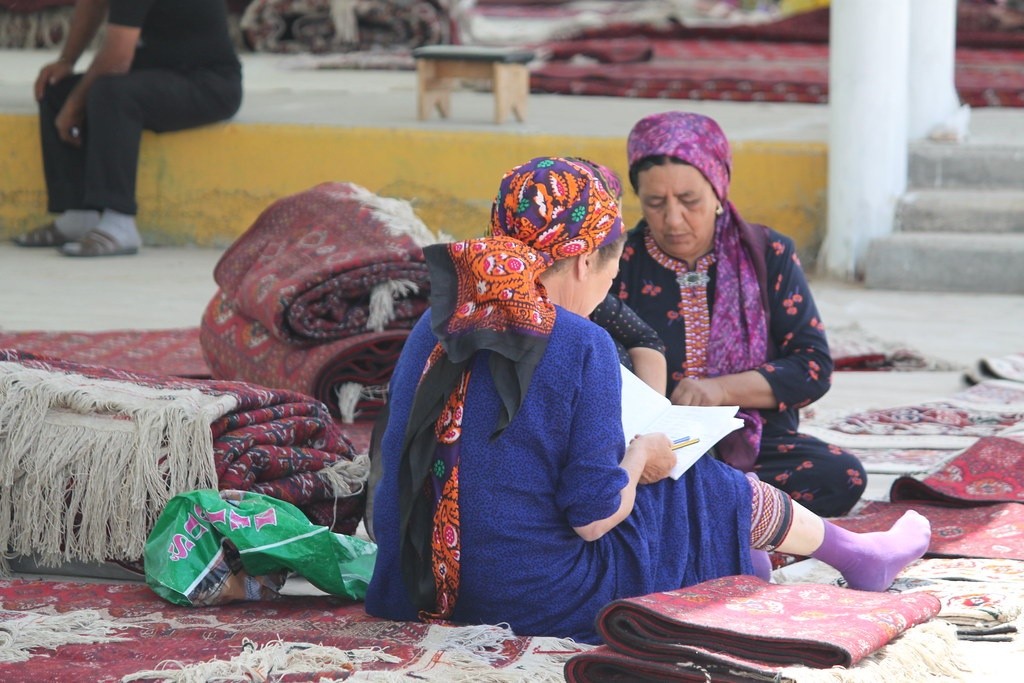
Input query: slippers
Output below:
<box><xmin>61</xmin><ymin>228</ymin><xmax>136</xmax><ymax>257</ymax></box>
<box><xmin>10</xmin><ymin>219</ymin><xmax>79</xmax><ymax>246</ymax></box>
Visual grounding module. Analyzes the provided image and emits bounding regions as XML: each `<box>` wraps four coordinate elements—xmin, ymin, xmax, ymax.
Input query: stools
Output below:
<box><xmin>413</xmin><ymin>43</ymin><xmax>534</xmax><ymax>123</ymax></box>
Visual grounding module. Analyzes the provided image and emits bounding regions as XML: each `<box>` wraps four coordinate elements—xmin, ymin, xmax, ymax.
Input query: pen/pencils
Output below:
<box><xmin>671</xmin><ymin>435</ymin><xmax>691</xmax><ymax>445</ymax></box>
<box><xmin>671</xmin><ymin>438</ymin><xmax>700</xmax><ymax>451</ymax></box>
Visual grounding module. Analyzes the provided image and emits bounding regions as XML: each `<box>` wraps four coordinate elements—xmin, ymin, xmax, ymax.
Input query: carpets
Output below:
<box><xmin>238</xmin><ymin>0</ymin><xmax>1024</xmax><ymax>108</ymax></box>
<box><xmin>0</xmin><ymin>179</ymin><xmax>1024</xmax><ymax>683</ymax></box>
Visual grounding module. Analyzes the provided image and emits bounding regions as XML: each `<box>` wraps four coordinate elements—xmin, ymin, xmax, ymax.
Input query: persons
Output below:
<box><xmin>590</xmin><ymin>299</ymin><xmax>671</xmax><ymax>400</ymax></box>
<box><xmin>363</xmin><ymin>152</ymin><xmax>934</xmax><ymax>643</ymax></box>
<box><xmin>616</xmin><ymin>111</ymin><xmax>867</xmax><ymax>516</ymax></box>
<box><xmin>8</xmin><ymin>0</ymin><xmax>245</xmax><ymax>260</ymax></box>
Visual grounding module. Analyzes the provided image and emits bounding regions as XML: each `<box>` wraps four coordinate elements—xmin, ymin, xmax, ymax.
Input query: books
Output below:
<box><xmin>616</xmin><ymin>359</ymin><xmax>744</xmax><ymax>482</ymax></box>
